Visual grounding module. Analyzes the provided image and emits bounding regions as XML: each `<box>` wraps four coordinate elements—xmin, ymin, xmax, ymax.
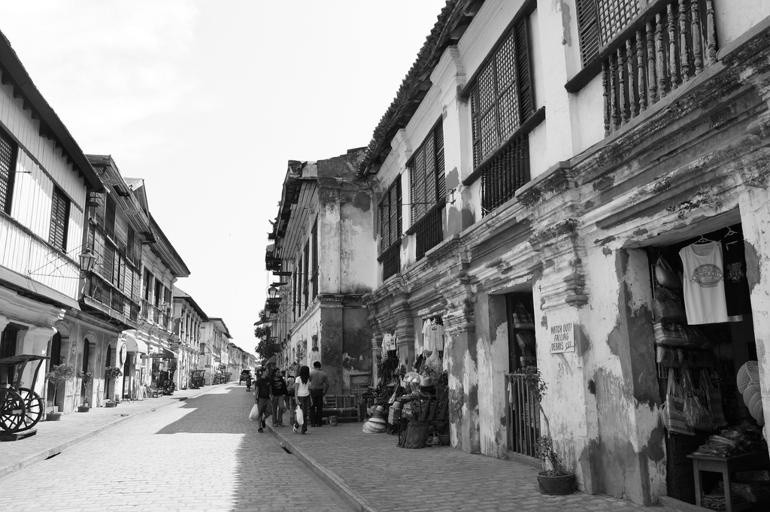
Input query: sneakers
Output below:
<box><xmin>261</xmin><ymin>420</ymin><xmax>265</xmax><ymax>428</ymax></box>
<box><xmin>292</xmin><ymin>423</ymin><xmax>298</xmax><ymax>433</ymax></box>
<box><xmin>257</xmin><ymin>427</ymin><xmax>263</xmax><ymax>433</ymax></box>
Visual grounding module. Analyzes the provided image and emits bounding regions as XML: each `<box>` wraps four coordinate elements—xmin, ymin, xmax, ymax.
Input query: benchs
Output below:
<box><xmin>321</xmin><ymin>394</ymin><xmax>361</xmax><ymax>424</ymax></box>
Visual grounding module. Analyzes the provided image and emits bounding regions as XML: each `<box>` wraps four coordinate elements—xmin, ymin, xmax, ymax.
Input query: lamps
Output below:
<box><xmin>77</xmin><ymin>242</ymin><xmax>98</xmax><ymax>280</ymax></box>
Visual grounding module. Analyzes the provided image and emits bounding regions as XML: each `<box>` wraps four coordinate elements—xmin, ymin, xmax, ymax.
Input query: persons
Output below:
<box><xmin>292</xmin><ymin>365</ymin><xmax>312</xmax><ymax>433</ymax></box>
<box><xmin>308</xmin><ymin>360</ymin><xmax>330</xmax><ymax>428</ymax></box>
<box><xmin>285</xmin><ymin>374</ymin><xmax>295</xmax><ymax>409</ymax></box>
<box><xmin>253</xmin><ymin>367</ymin><xmax>273</xmax><ymax>432</ymax></box>
<box><xmin>270</xmin><ymin>367</ymin><xmax>289</xmax><ymax>426</ymax></box>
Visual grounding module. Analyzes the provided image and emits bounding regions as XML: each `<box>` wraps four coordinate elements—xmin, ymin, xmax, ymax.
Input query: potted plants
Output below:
<box><xmin>44</xmin><ymin>360</ymin><xmax>74</xmax><ymax>422</ymax></box>
<box><xmin>104</xmin><ymin>365</ymin><xmax>121</xmax><ymax>408</ymax></box>
<box><xmin>76</xmin><ymin>370</ymin><xmax>92</xmax><ymax>413</ymax></box>
<box><xmin>510</xmin><ymin>367</ymin><xmax>579</xmax><ymax>496</ymax></box>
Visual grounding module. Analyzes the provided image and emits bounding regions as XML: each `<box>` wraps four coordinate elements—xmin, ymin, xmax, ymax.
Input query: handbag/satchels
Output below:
<box><xmin>295</xmin><ymin>405</ymin><xmax>304</xmax><ymax>425</ymax></box>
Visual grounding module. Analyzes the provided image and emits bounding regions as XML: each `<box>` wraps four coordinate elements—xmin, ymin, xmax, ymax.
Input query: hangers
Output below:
<box><xmin>686</xmin><ymin>232</ymin><xmax>718</xmax><ymax>255</ymax></box>
<box><xmin>718</xmin><ymin>225</ymin><xmax>740</xmax><ymax>247</ymax></box>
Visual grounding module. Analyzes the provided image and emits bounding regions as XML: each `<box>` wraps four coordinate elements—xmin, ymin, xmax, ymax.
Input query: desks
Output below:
<box><xmin>686</xmin><ymin>445</ymin><xmax>769</xmax><ymax>511</ymax></box>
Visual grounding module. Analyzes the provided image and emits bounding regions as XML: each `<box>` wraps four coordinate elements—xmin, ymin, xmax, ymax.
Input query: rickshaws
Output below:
<box><xmin>0</xmin><ymin>354</ymin><xmax>51</xmax><ymax>433</ymax></box>
<box><xmin>189</xmin><ymin>369</ymin><xmax>206</xmax><ymax>388</ymax></box>
<box><xmin>153</xmin><ymin>358</ymin><xmax>178</xmax><ymax>395</ymax></box>
